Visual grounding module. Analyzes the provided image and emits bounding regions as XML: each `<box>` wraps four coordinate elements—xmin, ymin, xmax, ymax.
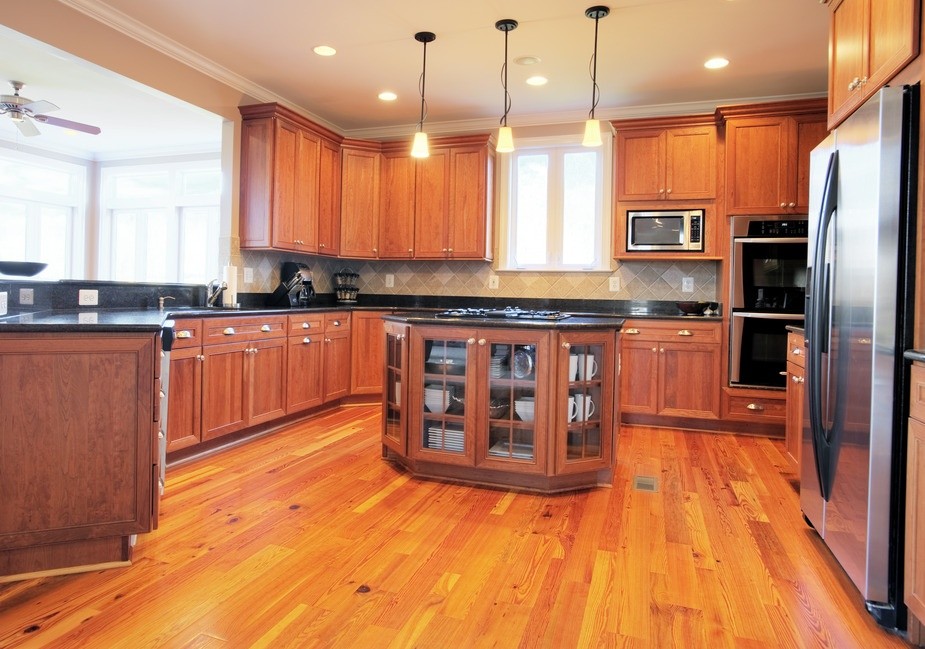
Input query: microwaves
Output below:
<box><xmin>626</xmin><ymin>208</ymin><xmax>704</xmax><ymax>253</ymax></box>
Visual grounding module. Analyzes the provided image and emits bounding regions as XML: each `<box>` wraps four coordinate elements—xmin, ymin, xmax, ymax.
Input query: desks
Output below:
<box><xmin>377</xmin><ymin>311</ymin><xmax>630</xmax><ymax>497</ymax></box>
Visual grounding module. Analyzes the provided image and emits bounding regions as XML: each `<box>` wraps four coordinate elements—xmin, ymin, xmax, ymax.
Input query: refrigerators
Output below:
<box><xmin>800</xmin><ymin>82</ymin><xmax>922</xmax><ymax>640</ymax></box>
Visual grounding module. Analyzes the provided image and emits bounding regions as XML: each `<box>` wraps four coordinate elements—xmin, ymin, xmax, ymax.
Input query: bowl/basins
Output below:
<box><xmin>675</xmin><ymin>302</ymin><xmax>710</xmax><ymax>316</ymax></box>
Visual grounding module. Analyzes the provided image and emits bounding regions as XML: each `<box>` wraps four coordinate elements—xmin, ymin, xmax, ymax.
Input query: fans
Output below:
<box><xmin>0</xmin><ymin>80</ymin><xmax>102</xmax><ymax>142</ymax></box>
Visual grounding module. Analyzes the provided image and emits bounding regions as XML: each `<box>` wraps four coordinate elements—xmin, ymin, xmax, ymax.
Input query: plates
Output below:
<box><xmin>394</xmin><ymin>343</ymin><xmax>537</xmax><ymax>461</ymax></box>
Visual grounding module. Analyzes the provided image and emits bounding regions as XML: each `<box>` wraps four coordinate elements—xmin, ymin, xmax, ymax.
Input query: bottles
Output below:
<box><xmin>334</xmin><ymin>272</ymin><xmax>360</xmax><ymax>300</ymax></box>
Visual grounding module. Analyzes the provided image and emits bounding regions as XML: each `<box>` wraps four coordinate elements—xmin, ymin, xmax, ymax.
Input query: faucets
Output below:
<box><xmin>204</xmin><ymin>277</ymin><xmax>229</xmax><ymax>308</ymax></box>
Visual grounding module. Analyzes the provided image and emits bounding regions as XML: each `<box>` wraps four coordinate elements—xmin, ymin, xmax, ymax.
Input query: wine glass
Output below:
<box><xmin>709</xmin><ymin>302</ymin><xmax>719</xmax><ymax>316</ymax></box>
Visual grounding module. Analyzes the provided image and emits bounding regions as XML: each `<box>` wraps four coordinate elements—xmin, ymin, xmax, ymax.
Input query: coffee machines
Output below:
<box><xmin>281</xmin><ymin>261</ymin><xmax>317</xmax><ymax>307</ymax></box>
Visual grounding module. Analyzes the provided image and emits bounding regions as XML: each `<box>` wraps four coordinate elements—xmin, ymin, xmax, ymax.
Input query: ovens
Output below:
<box><xmin>729</xmin><ymin>215</ymin><xmax>809</xmax><ymax>391</ymax></box>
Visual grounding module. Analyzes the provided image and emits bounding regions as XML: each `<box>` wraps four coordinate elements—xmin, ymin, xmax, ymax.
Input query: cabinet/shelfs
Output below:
<box><xmin>613</xmin><ymin>304</ymin><xmax>816</xmax><ymax>489</ymax></box>
<box><xmin>1</xmin><ymin>305</ymin><xmax>392</xmax><ymax>586</ymax></box>
<box><xmin>233</xmin><ymin>99</ymin><xmax>502</xmax><ymax>266</ymax></box>
<box><xmin>604</xmin><ymin>96</ymin><xmax>831</xmax><ymax>266</ymax></box>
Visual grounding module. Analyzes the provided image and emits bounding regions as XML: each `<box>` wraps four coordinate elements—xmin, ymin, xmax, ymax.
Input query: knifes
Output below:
<box><xmin>285</xmin><ymin>272</ymin><xmax>305</xmax><ymax>292</ymax></box>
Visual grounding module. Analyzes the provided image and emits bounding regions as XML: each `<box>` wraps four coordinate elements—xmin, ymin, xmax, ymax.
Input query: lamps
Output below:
<box><xmin>581</xmin><ymin>3</ymin><xmax>614</xmax><ymax>147</ymax></box>
<box><xmin>408</xmin><ymin>30</ymin><xmax>434</xmax><ymax>159</ymax></box>
<box><xmin>489</xmin><ymin>17</ymin><xmax>520</xmax><ymax>152</ymax></box>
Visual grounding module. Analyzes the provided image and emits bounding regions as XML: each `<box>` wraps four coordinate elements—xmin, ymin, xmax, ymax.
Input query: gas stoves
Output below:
<box><xmin>434</xmin><ymin>305</ymin><xmax>570</xmax><ymax>321</ymax></box>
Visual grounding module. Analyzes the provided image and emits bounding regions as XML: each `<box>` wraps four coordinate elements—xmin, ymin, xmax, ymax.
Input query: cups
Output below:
<box><xmin>568</xmin><ymin>395</ymin><xmax>578</xmax><ymax>423</ymax></box>
<box><xmin>569</xmin><ymin>353</ymin><xmax>578</xmax><ymax>383</ymax></box>
<box><xmin>578</xmin><ymin>353</ymin><xmax>597</xmax><ymax>382</ymax></box>
<box><xmin>573</xmin><ymin>393</ymin><xmax>594</xmax><ymax>423</ymax></box>
